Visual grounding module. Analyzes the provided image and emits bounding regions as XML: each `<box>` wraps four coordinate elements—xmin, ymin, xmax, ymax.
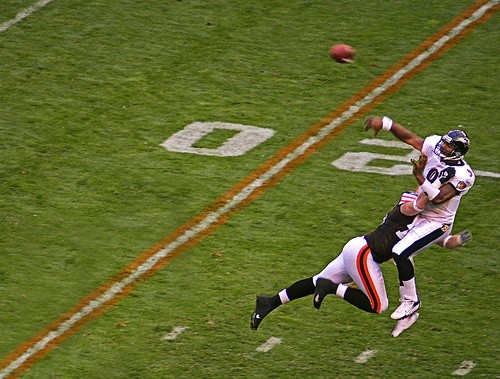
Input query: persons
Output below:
<box><xmin>364</xmin><ymin>114</ymin><xmax>476</xmax><ymax>338</ymax></box>
<box><xmin>250</xmin><ymin>167</ymin><xmax>473</xmax><ymax>330</ymax></box>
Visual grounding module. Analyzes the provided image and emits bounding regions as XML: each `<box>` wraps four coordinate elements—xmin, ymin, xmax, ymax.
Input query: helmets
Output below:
<box><xmin>443</xmin><ymin>129</ymin><xmax>471</xmax><ymax>157</ymax></box>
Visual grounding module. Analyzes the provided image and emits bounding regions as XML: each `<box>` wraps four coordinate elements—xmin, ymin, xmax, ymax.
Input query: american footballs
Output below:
<box><xmin>329</xmin><ymin>43</ymin><xmax>356</xmax><ymax>64</ymax></box>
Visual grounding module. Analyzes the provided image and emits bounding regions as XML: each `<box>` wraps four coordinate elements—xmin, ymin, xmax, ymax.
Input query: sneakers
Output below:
<box><xmin>391</xmin><ymin>312</ymin><xmax>420</xmax><ymax>338</ymax></box>
<box><xmin>313</xmin><ymin>278</ymin><xmax>332</xmax><ymax>309</ymax></box>
<box><xmin>390</xmin><ymin>298</ymin><xmax>422</xmax><ymax>320</ymax></box>
<box><xmin>250</xmin><ymin>296</ymin><xmax>272</xmax><ymax>330</ymax></box>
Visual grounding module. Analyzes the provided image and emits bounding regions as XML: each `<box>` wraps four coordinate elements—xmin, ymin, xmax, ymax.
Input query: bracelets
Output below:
<box><xmin>381</xmin><ymin>115</ymin><xmax>392</xmax><ymax>131</ymax></box>
